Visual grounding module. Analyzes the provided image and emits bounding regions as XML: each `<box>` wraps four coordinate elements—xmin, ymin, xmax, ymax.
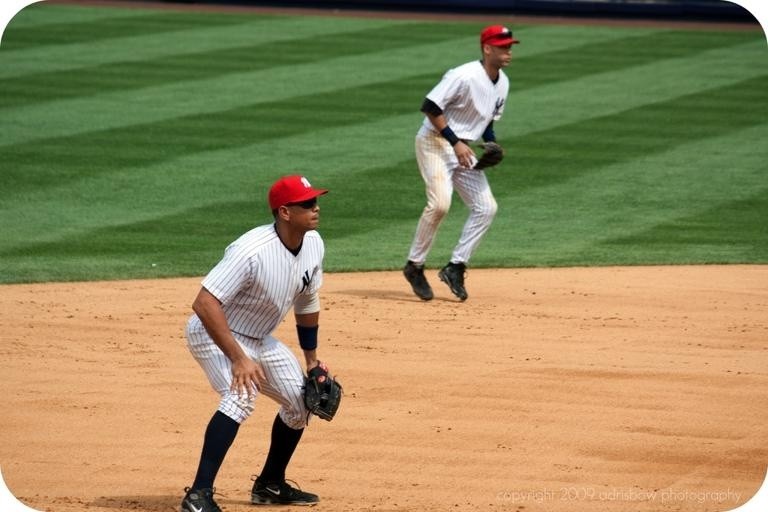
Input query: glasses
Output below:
<box><xmin>285</xmin><ymin>196</ymin><xmax>319</xmax><ymax>210</ymax></box>
<box><xmin>486</xmin><ymin>30</ymin><xmax>515</xmax><ymax>40</ymax></box>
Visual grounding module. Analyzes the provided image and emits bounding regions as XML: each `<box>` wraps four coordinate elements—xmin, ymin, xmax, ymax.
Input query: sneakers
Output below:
<box><xmin>250</xmin><ymin>473</ymin><xmax>320</xmax><ymax>507</ymax></box>
<box><xmin>180</xmin><ymin>485</ymin><xmax>223</xmax><ymax>512</ymax></box>
<box><xmin>402</xmin><ymin>259</ymin><xmax>434</xmax><ymax>301</ymax></box>
<box><xmin>438</xmin><ymin>260</ymin><xmax>469</xmax><ymax>302</ymax></box>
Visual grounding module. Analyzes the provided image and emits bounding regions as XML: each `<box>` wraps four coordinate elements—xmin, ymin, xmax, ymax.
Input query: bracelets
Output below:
<box><xmin>296</xmin><ymin>322</ymin><xmax>318</xmax><ymax>350</ymax></box>
<box><xmin>440</xmin><ymin>125</ymin><xmax>459</xmax><ymax>147</ymax></box>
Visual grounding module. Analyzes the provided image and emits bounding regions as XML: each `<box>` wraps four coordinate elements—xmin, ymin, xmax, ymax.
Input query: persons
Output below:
<box><xmin>401</xmin><ymin>24</ymin><xmax>522</xmax><ymax>303</ymax></box>
<box><xmin>178</xmin><ymin>174</ymin><xmax>341</xmax><ymax>511</ymax></box>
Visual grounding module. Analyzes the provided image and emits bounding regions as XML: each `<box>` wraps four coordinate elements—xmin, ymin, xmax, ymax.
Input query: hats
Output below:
<box><xmin>269</xmin><ymin>174</ymin><xmax>330</xmax><ymax>209</ymax></box>
<box><xmin>479</xmin><ymin>24</ymin><xmax>521</xmax><ymax>48</ymax></box>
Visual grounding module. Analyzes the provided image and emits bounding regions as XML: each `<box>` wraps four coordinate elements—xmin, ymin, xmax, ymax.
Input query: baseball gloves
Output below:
<box><xmin>473</xmin><ymin>141</ymin><xmax>505</xmax><ymax>169</ymax></box>
<box><xmin>304</xmin><ymin>360</ymin><xmax>341</xmax><ymax>421</ymax></box>
